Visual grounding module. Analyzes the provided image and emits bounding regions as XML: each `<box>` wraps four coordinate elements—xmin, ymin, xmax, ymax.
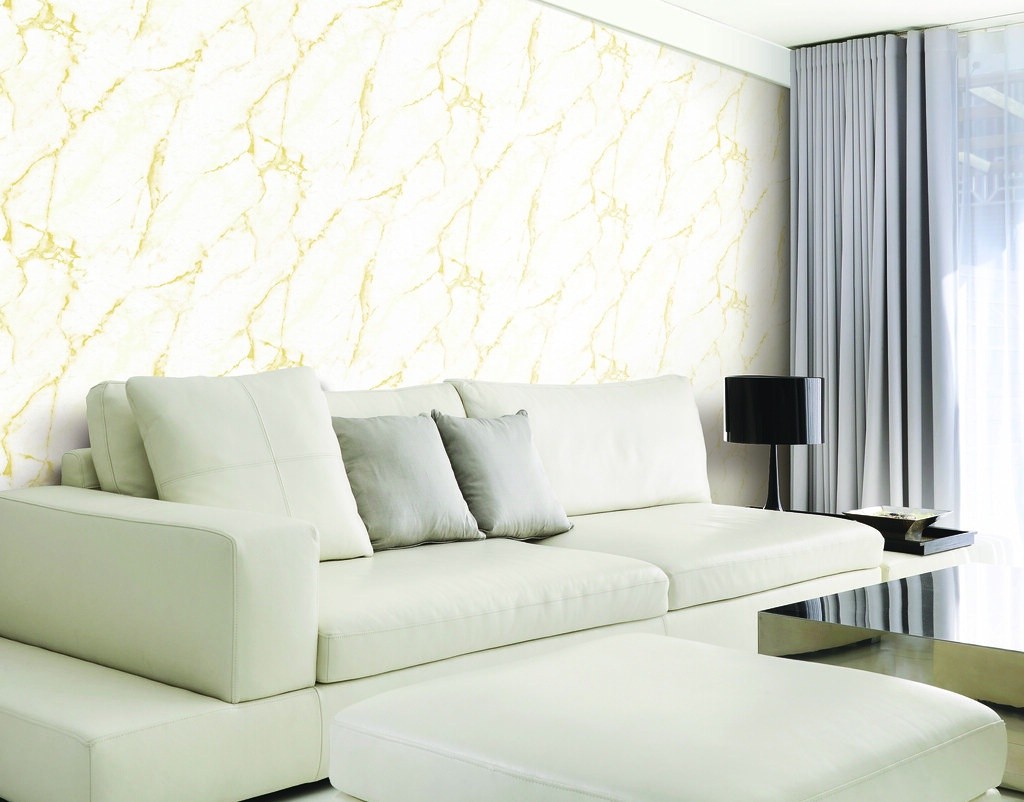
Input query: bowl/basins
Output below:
<box><xmin>841</xmin><ymin>504</ymin><xmax>954</xmax><ymax>539</ymax></box>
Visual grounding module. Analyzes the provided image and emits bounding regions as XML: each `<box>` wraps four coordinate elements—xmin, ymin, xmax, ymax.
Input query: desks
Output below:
<box><xmin>881</xmin><ymin>544</ymin><xmax>977</xmax><ymax>583</ymax></box>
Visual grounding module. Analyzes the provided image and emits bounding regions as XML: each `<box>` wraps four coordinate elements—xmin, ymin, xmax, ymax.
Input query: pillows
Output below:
<box><xmin>125</xmin><ymin>367</ymin><xmax>573</xmax><ymax>563</ymax></box>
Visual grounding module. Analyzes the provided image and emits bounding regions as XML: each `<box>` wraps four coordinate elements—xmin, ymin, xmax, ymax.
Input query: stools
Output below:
<box><xmin>329</xmin><ymin>633</ymin><xmax>1005</xmax><ymax>802</ymax></box>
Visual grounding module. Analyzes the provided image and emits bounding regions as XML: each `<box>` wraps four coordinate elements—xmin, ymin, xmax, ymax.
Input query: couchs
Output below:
<box><xmin>0</xmin><ymin>368</ymin><xmax>885</xmax><ymax>802</ymax></box>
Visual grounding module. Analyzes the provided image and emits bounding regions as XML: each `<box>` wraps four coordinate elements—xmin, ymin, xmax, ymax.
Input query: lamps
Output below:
<box><xmin>724</xmin><ymin>375</ymin><xmax>825</xmax><ymax>511</ymax></box>
